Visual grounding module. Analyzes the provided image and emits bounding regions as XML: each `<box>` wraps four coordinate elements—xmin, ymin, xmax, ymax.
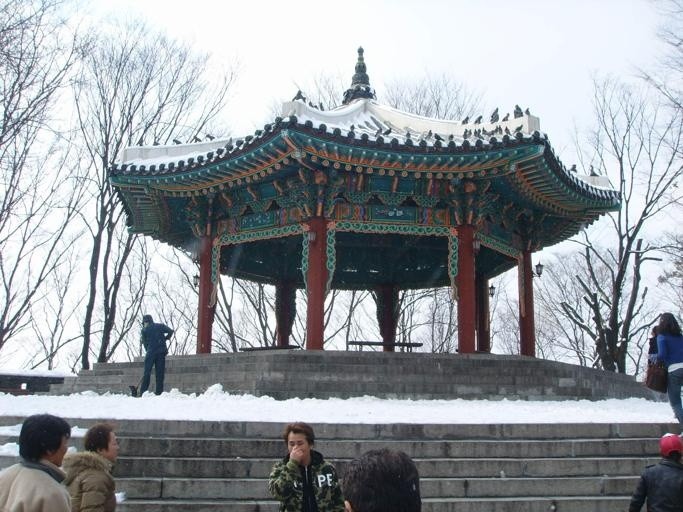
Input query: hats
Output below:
<box><xmin>142</xmin><ymin>314</ymin><xmax>153</xmax><ymax>323</ymax></box>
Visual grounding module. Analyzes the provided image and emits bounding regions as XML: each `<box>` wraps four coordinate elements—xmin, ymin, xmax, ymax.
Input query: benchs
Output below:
<box><xmin>348</xmin><ymin>340</ymin><xmax>424</xmax><ymax>352</ymax></box>
<box><xmin>238</xmin><ymin>344</ymin><xmax>301</xmax><ymax>352</ymax></box>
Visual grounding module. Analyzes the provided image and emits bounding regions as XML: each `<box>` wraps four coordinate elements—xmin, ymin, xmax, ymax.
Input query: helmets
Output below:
<box><xmin>660</xmin><ymin>433</ymin><xmax>682</xmax><ymax>457</ymax></box>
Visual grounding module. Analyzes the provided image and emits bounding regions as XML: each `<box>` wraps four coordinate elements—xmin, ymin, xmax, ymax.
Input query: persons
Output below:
<box><xmin>0</xmin><ymin>414</ymin><xmax>72</xmax><ymax>512</ymax></box>
<box><xmin>629</xmin><ymin>432</ymin><xmax>683</xmax><ymax>511</ymax></box>
<box><xmin>341</xmin><ymin>448</ymin><xmax>422</xmax><ymax>512</ymax></box>
<box><xmin>139</xmin><ymin>315</ymin><xmax>174</xmax><ymax>397</ymax></box>
<box><xmin>63</xmin><ymin>425</ymin><xmax>119</xmax><ymax>512</ymax></box>
<box><xmin>269</xmin><ymin>422</ymin><xmax>345</xmax><ymax>512</ymax></box>
<box><xmin>648</xmin><ymin>313</ymin><xmax>683</xmax><ymax>437</ymax></box>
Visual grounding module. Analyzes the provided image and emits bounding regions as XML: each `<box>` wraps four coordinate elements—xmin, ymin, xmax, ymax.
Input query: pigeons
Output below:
<box><xmin>375</xmin><ymin>127</ymin><xmax>382</xmax><ymax>137</ymax></box>
<box><xmin>434</xmin><ymin>133</ymin><xmax>445</xmax><ymax>142</ymax></box>
<box><xmin>382</xmin><ymin>128</ymin><xmax>391</xmax><ymax>137</ymax></box>
<box><xmin>513</xmin><ymin>125</ymin><xmax>523</xmax><ymax>134</ymax></box>
<box><xmin>406</xmin><ymin>131</ymin><xmax>411</xmax><ymax>138</ymax></box>
<box><xmin>525</xmin><ymin>108</ymin><xmax>530</xmax><ymax>116</ymax></box>
<box><xmin>224</xmin><ymin>137</ymin><xmax>233</xmax><ymax>148</ymax></box>
<box><xmin>350</xmin><ymin>124</ymin><xmax>354</xmax><ymax>132</ymax></box>
<box><xmin>448</xmin><ymin>134</ymin><xmax>454</xmax><ymax>141</ymax></box>
<box><xmin>463</xmin><ymin>125</ymin><xmax>503</xmax><ymax>140</ymax></box>
<box><xmin>504</xmin><ymin>126</ymin><xmax>512</xmax><ymax>137</ymax></box>
<box><xmin>425</xmin><ymin>130</ymin><xmax>432</xmax><ymax>140</ymax></box>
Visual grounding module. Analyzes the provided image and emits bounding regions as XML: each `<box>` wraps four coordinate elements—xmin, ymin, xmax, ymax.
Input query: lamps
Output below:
<box><xmin>489</xmin><ymin>283</ymin><xmax>496</xmax><ymax>297</ymax></box>
<box><xmin>192</xmin><ymin>272</ymin><xmax>200</xmax><ymax>289</ymax></box>
<box><xmin>532</xmin><ymin>260</ymin><xmax>545</xmax><ymax>279</ymax></box>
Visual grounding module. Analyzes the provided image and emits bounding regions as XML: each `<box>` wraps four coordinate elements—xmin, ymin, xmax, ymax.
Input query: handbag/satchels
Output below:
<box><xmin>645</xmin><ymin>365</ymin><xmax>667</xmax><ymax>392</ymax></box>
